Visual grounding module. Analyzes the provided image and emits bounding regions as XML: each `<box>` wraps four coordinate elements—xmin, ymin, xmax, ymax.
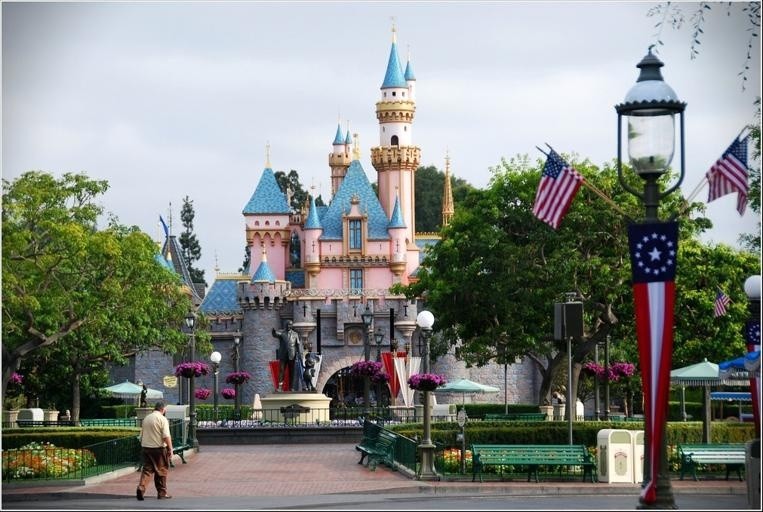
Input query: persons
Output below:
<box><xmin>302</xmin><ymin>352</ymin><xmax>317</xmax><ymax>392</ymax></box>
<box><xmin>139</xmin><ymin>384</ymin><xmax>147</xmax><ymax>408</ymax></box>
<box><xmin>271</xmin><ymin>319</ymin><xmax>303</xmax><ymax>393</ymax></box>
<box><xmin>551</xmin><ymin>385</ymin><xmax>565</xmax><ymax>406</ymax></box>
<box><xmin>135</xmin><ymin>399</ymin><xmax>173</xmax><ymax>500</ymax></box>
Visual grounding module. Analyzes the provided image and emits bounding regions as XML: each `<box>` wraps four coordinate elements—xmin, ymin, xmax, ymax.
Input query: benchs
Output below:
<box><xmin>136</xmin><ymin>433</ymin><xmax>190</xmax><ymax>472</ymax></box>
<box><xmin>79</xmin><ymin>418</ymin><xmax>135</xmax><ymax>427</ymax></box>
<box><xmin>481</xmin><ymin>413</ymin><xmax>547</xmax><ymax>421</ymax></box>
<box><xmin>679</xmin><ymin>442</ymin><xmax>745</xmax><ymax>482</ymax></box>
<box><xmin>355</xmin><ymin>426</ymin><xmax>398</xmax><ymax>471</ymax></box>
<box><xmin>471</xmin><ymin>442</ymin><xmax>596</xmax><ymax>482</ymax></box>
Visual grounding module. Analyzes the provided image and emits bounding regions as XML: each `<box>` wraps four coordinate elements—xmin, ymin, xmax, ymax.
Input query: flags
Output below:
<box><xmin>547</xmin><ymin>149</ymin><xmax>586</xmax><ymax>184</ymax></box>
<box><xmin>712</xmin><ymin>285</ymin><xmax>732</xmax><ymax>320</ymax></box>
<box><xmin>705</xmin><ymin>137</ymin><xmax>741</xmax><ymax>204</ymax></box>
<box><xmin>716</xmin><ymin>135</ymin><xmax>751</xmax><ymax>216</ymax></box>
<box><xmin>530</xmin><ymin>157</ymin><xmax>584</xmax><ymax>231</ymax></box>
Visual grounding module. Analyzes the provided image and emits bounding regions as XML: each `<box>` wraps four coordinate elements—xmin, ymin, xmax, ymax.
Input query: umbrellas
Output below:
<box><xmin>717</xmin><ymin>350</ymin><xmax>761</xmax><ymax>380</ymax></box>
<box><xmin>665</xmin><ymin>356</ymin><xmax>752</xmax><ymax>443</ymax></box>
<box><xmin>433</xmin><ymin>376</ymin><xmax>500</xmax><ymax>473</ymax></box>
<box><xmin>100</xmin><ymin>378</ymin><xmax>163</xmax><ymax>418</ymax></box>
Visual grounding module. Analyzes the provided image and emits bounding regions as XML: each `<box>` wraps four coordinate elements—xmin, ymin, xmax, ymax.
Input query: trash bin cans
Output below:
<box><xmin>433</xmin><ymin>404</ymin><xmax>456</xmax><ymax>415</ymax></box>
<box><xmin>17</xmin><ymin>408</ymin><xmax>44</xmax><ymax>426</ymax></box>
<box><xmin>597</xmin><ymin>428</ymin><xmax>645</xmax><ymax>483</ymax></box>
<box><xmin>164</xmin><ymin>404</ymin><xmax>190</xmax><ymax>445</ymax></box>
<box><xmin>745</xmin><ymin>438</ymin><xmax>761</xmax><ymax>509</ymax></box>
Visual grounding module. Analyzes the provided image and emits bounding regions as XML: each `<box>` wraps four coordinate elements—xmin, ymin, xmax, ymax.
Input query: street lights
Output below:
<box><xmin>360</xmin><ymin>300</ymin><xmax>376</xmax><ymax>419</ymax></box>
<box><xmin>612</xmin><ymin>41</ymin><xmax>687</xmax><ymax>510</ymax></box>
<box><xmin>185</xmin><ymin>308</ymin><xmax>199</xmax><ymax>452</ymax></box>
<box><xmin>210</xmin><ymin>351</ymin><xmax>222</xmax><ymax>423</ymax></box>
<box><xmin>743</xmin><ymin>274</ymin><xmax>762</xmax><ymax>319</ymax></box>
<box><xmin>231</xmin><ymin>329</ymin><xmax>243</xmax><ymax>421</ymax></box>
<box><xmin>412</xmin><ymin>310</ymin><xmax>443</xmax><ymax>480</ymax></box>
<box><xmin>373</xmin><ymin>328</ymin><xmax>385</xmax><ymax>419</ymax></box>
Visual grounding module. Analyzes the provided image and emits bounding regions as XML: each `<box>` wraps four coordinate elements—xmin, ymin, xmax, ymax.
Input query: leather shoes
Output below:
<box><xmin>159</xmin><ymin>494</ymin><xmax>171</xmax><ymax>500</ymax></box>
<box><xmin>137</xmin><ymin>486</ymin><xmax>145</xmax><ymax>500</ymax></box>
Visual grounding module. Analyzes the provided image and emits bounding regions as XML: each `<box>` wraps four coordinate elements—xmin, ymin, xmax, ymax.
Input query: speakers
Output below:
<box><xmin>316</xmin><ymin>308</ymin><xmax>321</xmax><ymax>355</ymax></box>
<box><xmin>389</xmin><ymin>308</ymin><xmax>394</xmax><ymax>353</ymax></box>
